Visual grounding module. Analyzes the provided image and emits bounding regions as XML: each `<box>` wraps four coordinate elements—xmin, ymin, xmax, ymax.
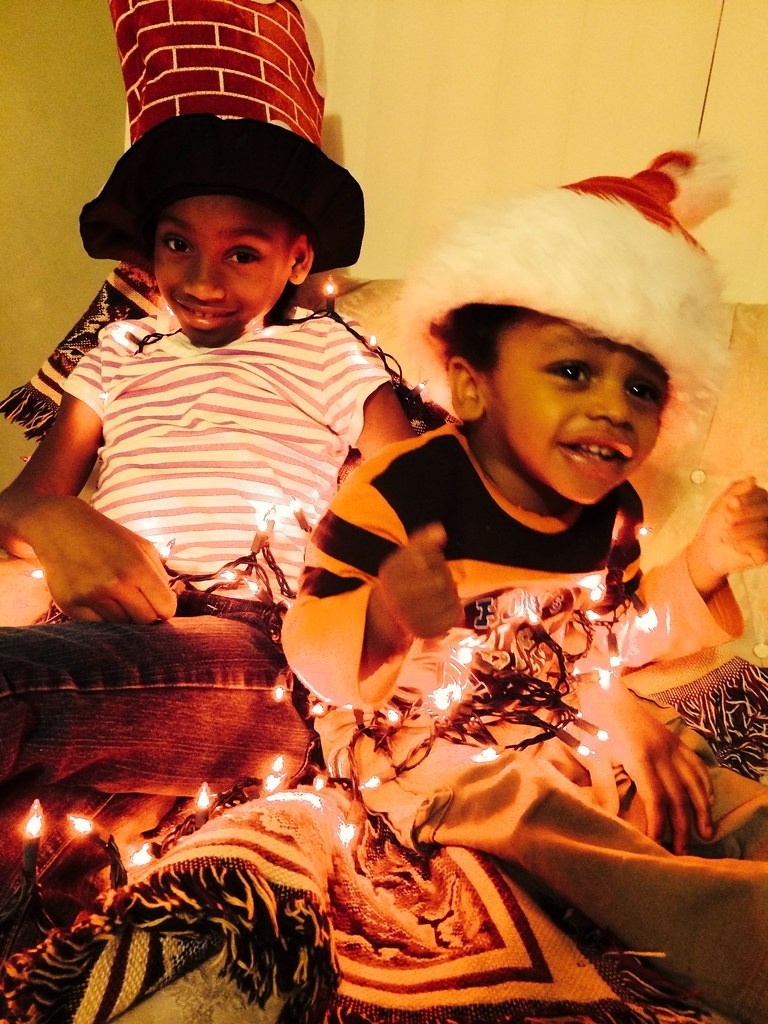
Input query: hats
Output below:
<box><xmin>395</xmin><ymin>152</ymin><xmax>730</xmax><ymax>404</ymax></box>
<box><xmin>79</xmin><ymin>0</ymin><xmax>366</xmax><ymax>275</ymax></box>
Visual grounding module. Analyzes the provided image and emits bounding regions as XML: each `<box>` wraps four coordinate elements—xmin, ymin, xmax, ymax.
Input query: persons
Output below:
<box><xmin>278</xmin><ymin>143</ymin><xmax>768</xmax><ymax>1024</ymax></box>
<box><xmin>0</xmin><ymin>114</ymin><xmax>716</xmax><ymax>972</ymax></box>
